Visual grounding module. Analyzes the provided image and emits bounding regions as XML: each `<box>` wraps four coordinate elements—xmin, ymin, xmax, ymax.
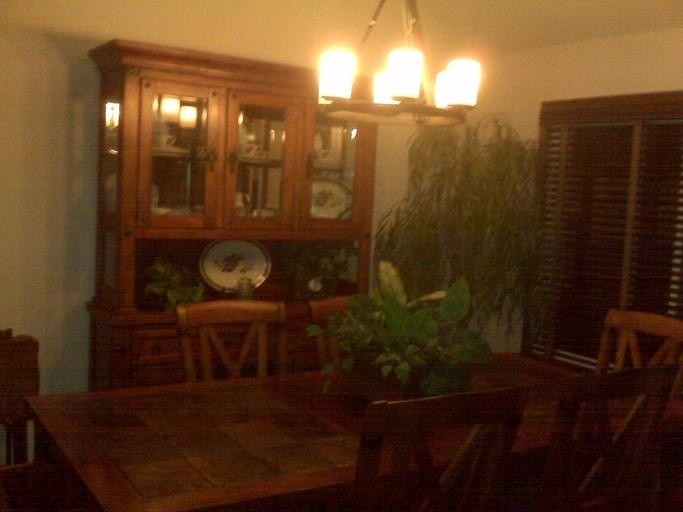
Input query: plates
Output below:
<box><xmin>310</xmin><ymin>174</ymin><xmax>352</xmax><ymax>219</ymax></box>
<box><xmin>198</xmin><ymin>238</ymin><xmax>274</xmax><ymax>295</ymax></box>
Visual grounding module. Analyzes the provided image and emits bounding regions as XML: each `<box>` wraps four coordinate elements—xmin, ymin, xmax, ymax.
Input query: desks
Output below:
<box><xmin>24</xmin><ymin>353</ymin><xmax>683</xmax><ymax>511</ymax></box>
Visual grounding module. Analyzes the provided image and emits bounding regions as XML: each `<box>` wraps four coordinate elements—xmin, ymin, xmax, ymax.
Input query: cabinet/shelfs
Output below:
<box><xmin>84</xmin><ymin>38</ymin><xmax>378</xmax><ymax>390</ymax></box>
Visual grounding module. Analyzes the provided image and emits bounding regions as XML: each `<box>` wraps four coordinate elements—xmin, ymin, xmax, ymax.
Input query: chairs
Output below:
<box><xmin>536</xmin><ymin>363</ymin><xmax>682</xmax><ymax>510</ymax></box>
<box><xmin>308</xmin><ymin>300</ymin><xmax>351</xmax><ymax>368</ymax></box>
<box><xmin>598</xmin><ymin>311</ymin><xmax>683</xmax><ymax>372</ymax></box>
<box><xmin>176</xmin><ymin>299</ymin><xmax>290</xmax><ymax>380</ymax></box>
<box><xmin>353</xmin><ymin>381</ymin><xmax>529</xmax><ymax>510</ymax></box>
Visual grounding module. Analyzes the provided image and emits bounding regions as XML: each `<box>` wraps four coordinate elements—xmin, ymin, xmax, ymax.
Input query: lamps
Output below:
<box><xmin>317</xmin><ymin>0</ymin><xmax>486</xmax><ymax>131</ymax></box>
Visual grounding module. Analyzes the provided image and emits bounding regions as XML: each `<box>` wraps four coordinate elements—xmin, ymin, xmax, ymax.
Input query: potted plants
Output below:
<box><xmin>305</xmin><ymin>256</ymin><xmax>494</xmax><ymax>433</ymax></box>
